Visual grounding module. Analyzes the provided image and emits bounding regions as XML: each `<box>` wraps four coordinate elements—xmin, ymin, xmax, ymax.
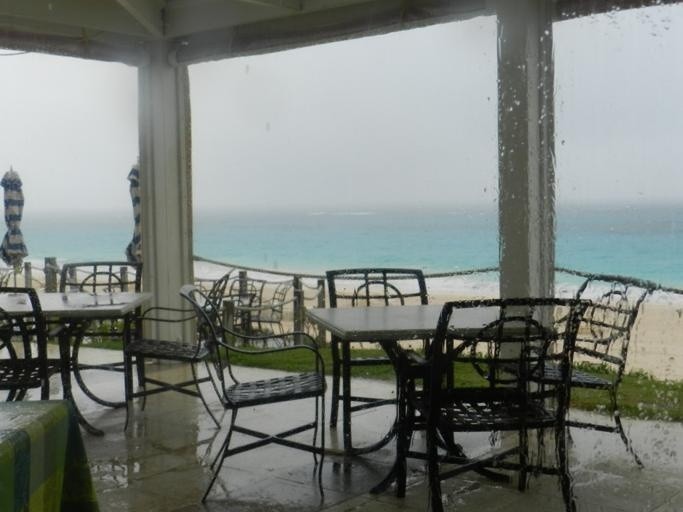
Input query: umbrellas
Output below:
<box><xmin>0</xmin><ymin>164</ymin><xmax>29</xmax><ymax>287</ymax></box>
<box><xmin>125</xmin><ymin>155</ymin><xmax>143</xmax><ymax>270</ymax></box>
